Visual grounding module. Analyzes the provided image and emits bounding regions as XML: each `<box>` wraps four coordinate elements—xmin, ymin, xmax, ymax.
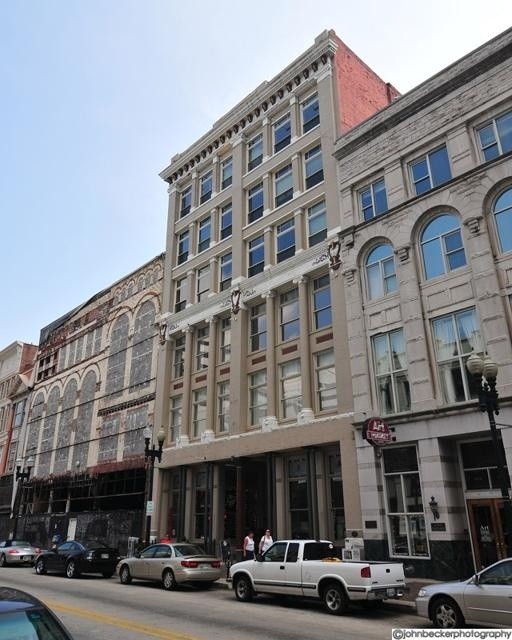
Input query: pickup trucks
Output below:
<box><xmin>228</xmin><ymin>539</ymin><xmax>409</xmax><ymax>615</ymax></box>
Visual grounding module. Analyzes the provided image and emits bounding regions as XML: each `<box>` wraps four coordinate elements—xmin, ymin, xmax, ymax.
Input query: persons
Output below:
<box><xmin>180</xmin><ymin>536</ymin><xmax>186</xmax><ymax>543</ymax></box>
<box><xmin>243</xmin><ymin>530</ymin><xmax>255</xmax><ymax>560</ymax></box>
<box><xmin>259</xmin><ymin>528</ymin><xmax>274</xmax><ymax>560</ymax></box>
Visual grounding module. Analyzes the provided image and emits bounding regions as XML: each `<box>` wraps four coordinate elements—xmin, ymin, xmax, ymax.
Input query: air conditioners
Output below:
<box><xmin>346</xmin><ymin>529</ymin><xmax>363</xmax><ymax>539</ymax></box>
<box><xmin>342</xmin><ymin>548</ymin><xmax>365</xmax><ymax>561</ymax></box>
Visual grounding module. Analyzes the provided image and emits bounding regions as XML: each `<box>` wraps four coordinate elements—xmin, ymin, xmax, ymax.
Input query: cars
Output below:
<box><xmin>0</xmin><ymin>540</ymin><xmax>38</xmax><ymax>567</ymax></box>
<box><xmin>416</xmin><ymin>559</ymin><xmax>511</xmax><ymax>629</ymax></box>
<box><xmin>35</xmin><ymin>540</ymin><xmax>120</xmax><ymax>579</ymax></box>
<box><xmin>116</xmin><ymin>543</ymin><xmax>222</xmax><ymax>590</ymax></box>
<box><xmin>1</xmin><ymin>587</ymin><xmax>72</xmax><ymax>639</ymax></box>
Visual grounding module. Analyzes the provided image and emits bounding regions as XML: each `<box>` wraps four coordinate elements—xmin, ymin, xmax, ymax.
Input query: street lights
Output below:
<box><xmin>467</xmin><ymin>346</ymin><xmax>512</xmax><ymax>556</ymax></box>
<box><xmin>13</xmin><ymin>454</ymin><xmax>35</xmax><ymax>540</ymax></box>
<box><xmin>144</xmin><ymin>423</ymin><xmax>166</xmax><ymax>545</ymax></box>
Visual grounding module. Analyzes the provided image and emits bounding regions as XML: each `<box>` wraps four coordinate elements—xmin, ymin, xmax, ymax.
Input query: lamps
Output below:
<box><xmin>429</xmin><ymin>494</ymin><xmax>440</xmax><ymax>521</ymax></box>
